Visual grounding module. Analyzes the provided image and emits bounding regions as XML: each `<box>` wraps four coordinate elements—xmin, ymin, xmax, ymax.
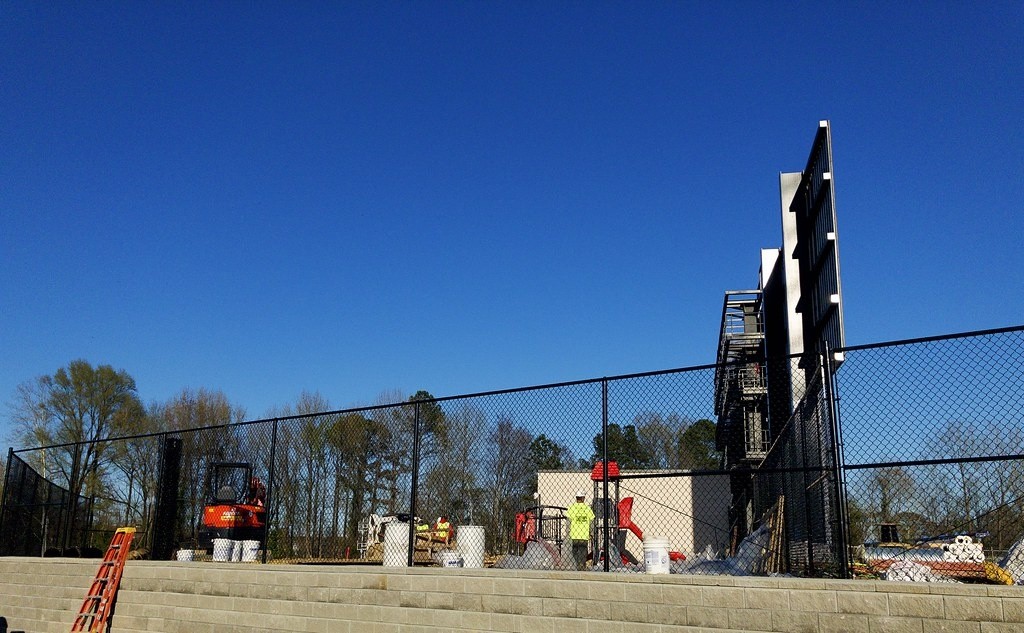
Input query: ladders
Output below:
<box><xmin>69</xmin><ymin>528</ymin><xmax>137</xmax><ymax>633</ymax></box>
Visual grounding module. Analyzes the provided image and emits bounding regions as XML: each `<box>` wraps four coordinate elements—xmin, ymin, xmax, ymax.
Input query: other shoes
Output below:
<box><xmin>577</xmin><ymin>566</ymin><xmax>587</xmax><ymax>570</ymax></box>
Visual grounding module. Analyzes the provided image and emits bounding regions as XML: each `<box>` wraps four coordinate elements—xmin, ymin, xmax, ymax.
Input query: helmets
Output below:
<box><xmin>574</xmin><ymin>490</ymin><xmax>586</xmax><ymax>497</ymax></box>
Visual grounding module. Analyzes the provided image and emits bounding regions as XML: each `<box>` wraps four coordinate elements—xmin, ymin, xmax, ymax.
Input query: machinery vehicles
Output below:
<box><xmin>199</xmin><ymin>462</ymin><xmax>268</xmax><ymax>552</ymax></box>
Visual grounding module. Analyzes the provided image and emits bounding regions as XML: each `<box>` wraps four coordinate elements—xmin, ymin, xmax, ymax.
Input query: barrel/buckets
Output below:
<box><xmin>212</xmin><ymin>539</ymin><xmax>233</xmax><ymax>562</ymax></box>
<box><xmin>383</xmin><ymin>524</ymin><xmax>414</xmax><ymax>567</ymax></box>
<box><xmin>443</xmin><ymin>554</ymin><xmax>460</xmax><ymax>567</ymax></box>
<box><xmin>242</xmin><ymin>540</ymin><xmax>260</xmax><ymax>562</ymax></box>
<box><xmin>458</xmin><ymin>526</ymin><xmax>485</xmax><ymax>568</ymax></box>
<box><xmin>643</xmin><ymin>536</ymin><xmax>671</xmax><ymax>575</ymax></box>
<box><xmin>231</xmin><ymin>541</ymin><xmax>242</xmax><ymax>562</ymax></box>
<box><xmin>177</xmin><ymin>549</ymin><xmax>194</xmax><ymax>561</ymax></box>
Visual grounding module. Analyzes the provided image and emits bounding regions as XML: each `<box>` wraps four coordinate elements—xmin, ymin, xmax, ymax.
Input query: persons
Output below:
<box><xmin>433</xmin><ymin>515</ymin><xmax>454</xmax><ymax>544</ymax></box>
<box><xmin>565</xmin><ymin>491</ymin><xmax>595</xmax><ymax>571</ymax></box>
<box><xmin>416</xmin><ymin>517</ymin><xmax>429</xmax><ymax>530</ymax></box>
